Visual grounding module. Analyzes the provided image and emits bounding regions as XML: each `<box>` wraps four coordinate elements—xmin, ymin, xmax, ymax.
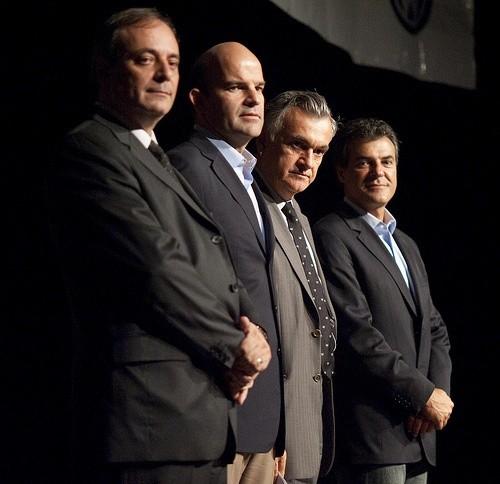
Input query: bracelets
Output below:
<box><xmin>255</xmin><ymin>324</ymin><xmax>269</xmax><ymax>341</ymax></box>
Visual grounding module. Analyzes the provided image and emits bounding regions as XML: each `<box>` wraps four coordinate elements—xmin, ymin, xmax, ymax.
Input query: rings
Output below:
<box><xmin>258</xmin><ymin>356</ymin><xmax>264</xmax><ymax>365</ymax></box>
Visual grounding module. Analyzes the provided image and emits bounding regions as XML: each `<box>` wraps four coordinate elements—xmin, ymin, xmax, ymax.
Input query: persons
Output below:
<box><xmin>62</xmin><ymin>8</ymin><xmax>272</xmax><ymax>484</ymax></box>
<box><xmin>313</xmin><ymin>118</ymin><xmax>455</xmax><ymax>484</ymax></box>
<box><xmin>250</xmin><ymin>90</ymin><xmax>337</xmax><ymax>484</ymax></box>
<box><xmin>166</xmin><ymin>42</ymin><xmax>287</xmax><ymax>484</ymax></box>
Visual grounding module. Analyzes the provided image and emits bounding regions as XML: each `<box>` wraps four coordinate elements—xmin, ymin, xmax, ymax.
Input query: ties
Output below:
<box><xmin>148</xmin><ymin>141</ymin><xmax>184</xmax><ymax>191</ymax></box>
<box><xmin>281</xmin><ymin>203</ymin><xmax>334</xmax><ymax>380</ymax></box>
